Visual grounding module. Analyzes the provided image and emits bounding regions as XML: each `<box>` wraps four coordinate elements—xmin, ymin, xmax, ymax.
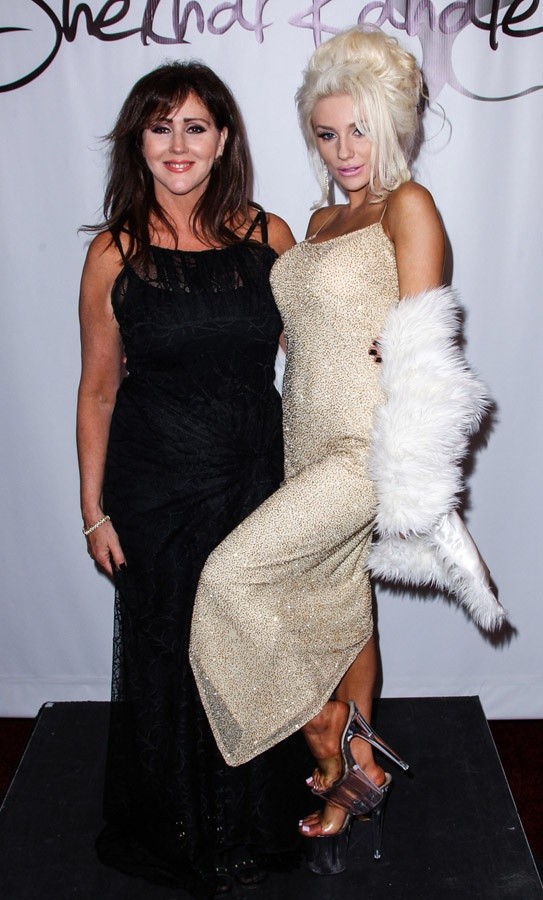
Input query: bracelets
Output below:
<box><xmin>82</xmin><ymin>514</ymin><xmax>111</xmax><ymax>536</ymax></box>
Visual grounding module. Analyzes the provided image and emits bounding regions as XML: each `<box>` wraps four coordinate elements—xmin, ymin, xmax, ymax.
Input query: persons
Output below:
<box><xmin>188</xmin><ymin>22</ymin><xmax>508</xmax><ymax>877</ymax></box>
<box><xmin>76</xmin><ymin>62</ymin><xmax>298</xmax><ymax>856</ymax></box>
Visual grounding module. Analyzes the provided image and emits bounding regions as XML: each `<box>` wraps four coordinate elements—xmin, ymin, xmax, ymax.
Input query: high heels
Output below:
<box><xmin>309</xmin><ymin>771</ymin><xmax>391</xmax><ymax>875</ymax></box>
<box><xmin>311</xmin><ymin>699</ymin><xmax>409</xmax><ymax>819</ymax></box>
